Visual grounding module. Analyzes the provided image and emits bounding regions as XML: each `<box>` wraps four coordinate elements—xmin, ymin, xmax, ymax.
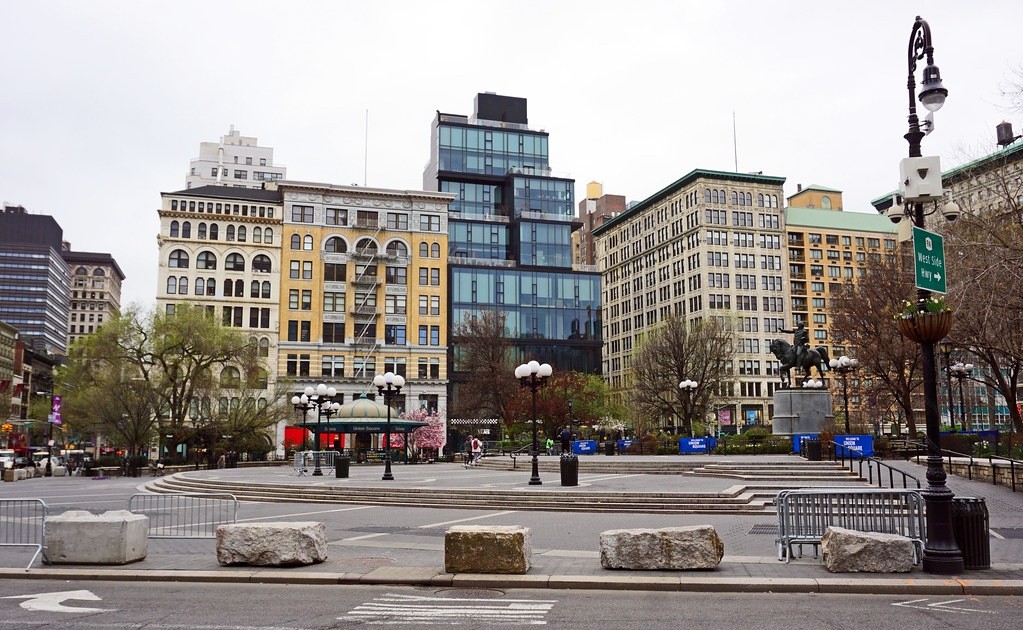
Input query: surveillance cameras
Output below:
<box><xmin>942</xmin><ymin>201</ymin><xmax>960</xmax><ymax>221</ymax></box>
<box><xmin>887</xmin><ymin>205</ymin><xmax>904</xmax><ymax>224</ymax></box>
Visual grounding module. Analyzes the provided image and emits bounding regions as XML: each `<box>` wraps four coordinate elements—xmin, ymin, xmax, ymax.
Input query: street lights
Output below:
<box><xmin>829</xmin><ymin>356</ymin><xmax>859</xmax><ymax>435</ymax></box>
<box><xmin>321</xmin><ymin>401</ymin><xmax>341</xmax><ymax>451</ymax></box>
<box><xmin>939</xmin><ymin>337</ymin><xmax>956</xmax><ymax>432</ymax></box>
<box><xmin>946</xmin><ymin>362</ymin><xmax>974</xmax><ymax>432</ymax></box>
<box><xmin>374</xmin><ymin>372</ymin><xmax>405</xmax><ymax>480</ymax></box>
<box><xmin>679</xmin><ymin>380</ymin><xmax>698</xmax><ymax>438</ymax></box>
<box><xmin>887</xmin><ymin>16</ymin><xmax>965</xmax><ymax>575</ymax></box>
<box><xmin>291</xmin><ymin>395</ymin><xmax>317</xmax><ymax>472</ymax></box>
<box><xmin>568</xmin><ymin>399</ymin><xmax>573</xmax><ymax>433</ymax></box>
<box><xmin>304</xmin><ymin>384</ymin><xmax>336</xmax><ymax>476</ymax></box>
<box><xmin>515</xmin><ymin>361</ymin><xmax>552</xmax><ymax>485</ymax></box>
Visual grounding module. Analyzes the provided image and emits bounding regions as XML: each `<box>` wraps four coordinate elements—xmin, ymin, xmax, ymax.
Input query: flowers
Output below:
<box><xmin>893</xmin><ymin>296</ymin><xmax>951</xmax><ymax>320</ymax></box>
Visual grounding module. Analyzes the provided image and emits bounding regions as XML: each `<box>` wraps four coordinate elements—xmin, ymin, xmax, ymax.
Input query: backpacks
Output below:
<box><xmin>473</xmin><ymin>439</ymin><xmax>478</xmax><ymax>449</ymax></box>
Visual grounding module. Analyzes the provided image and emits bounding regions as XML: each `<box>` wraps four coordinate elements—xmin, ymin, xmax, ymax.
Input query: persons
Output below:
<box><xmin>546</xmin><ymin>427</ymin><xmax>572</xmax><ymax>454</ymax></box>
<box><xmin>217</xmin><ymin>450</ymin><xmax>237</xmax><ymax>469</ymax></box>
<box><xmin>777</xmin><ymin>322</ymin><xmax>809</xmax><ymax>370</ymax></box>
<box><xmin>463</xmin><ymin>435</ymin><xmax>483</xmax><ymax>467</ymax></box>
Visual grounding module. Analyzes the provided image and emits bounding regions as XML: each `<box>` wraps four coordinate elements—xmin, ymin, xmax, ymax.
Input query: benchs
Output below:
<box><xmin>597</xmin><ymin>442</ymin><xmax>620</xmax><ymax>456</ymax></box>
<box><xmin>888</xmin><ymin>438</ymin><xmax>928</xmax><ymax>460</ymax></box>
<box><xmin>528</xmin><ymin>445</ymin><xmax>559</xmax><ymax>456</ymax></box>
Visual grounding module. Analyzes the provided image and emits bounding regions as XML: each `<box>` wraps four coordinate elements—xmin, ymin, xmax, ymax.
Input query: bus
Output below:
<box><xmin>0</xmin><ymin>448</ymin><xmax>84</xmax><ymax>470</ymax></box>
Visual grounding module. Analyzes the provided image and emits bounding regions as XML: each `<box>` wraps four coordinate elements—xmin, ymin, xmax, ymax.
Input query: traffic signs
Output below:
<box><xmin>912</xmin><ymin>226</ymin><xmax>947</xmax><ymax>295</ymax></box>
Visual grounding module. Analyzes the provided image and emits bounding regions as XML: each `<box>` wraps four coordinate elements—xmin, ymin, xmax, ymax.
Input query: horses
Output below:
<box><xmin>769</xmin><ymin>339</ymin><xmax>831</xmax><ymax>388</ymax></box>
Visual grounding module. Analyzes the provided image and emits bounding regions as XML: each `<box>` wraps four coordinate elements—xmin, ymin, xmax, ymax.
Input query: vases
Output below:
<box><xmin>896</xmin><ymin>311</ymin><xmax>954</xmax><ymax>344</ymax></box>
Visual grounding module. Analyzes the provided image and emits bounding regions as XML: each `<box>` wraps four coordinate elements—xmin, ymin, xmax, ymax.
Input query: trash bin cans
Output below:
<box><xmin>605</xmin><ymin>441</ymin><xmax>615</xmax><ymax>455</ymax></box>
<box><xmin>335</xmin><ymin>453</ymin><xmax>349</xmax><ymax>478</ymax></box>
<box><xmin>808</xmin><ymin>440</ymin><xmax>822</xmax><ymax>461</ymax></box>
<box><xmin>951</xmin><ymin>497</ymin><xmax>990</xmax><ymax>570</ymax></box>
<box><xmin>560</xmin><ymin>455</ymin><xmax>578</xmax><ymax>486</ymax></box>
<box><xmin>225</xmin><ymin>453</ymin><xmax>238</xmax><ymax>468</ymax></box>
